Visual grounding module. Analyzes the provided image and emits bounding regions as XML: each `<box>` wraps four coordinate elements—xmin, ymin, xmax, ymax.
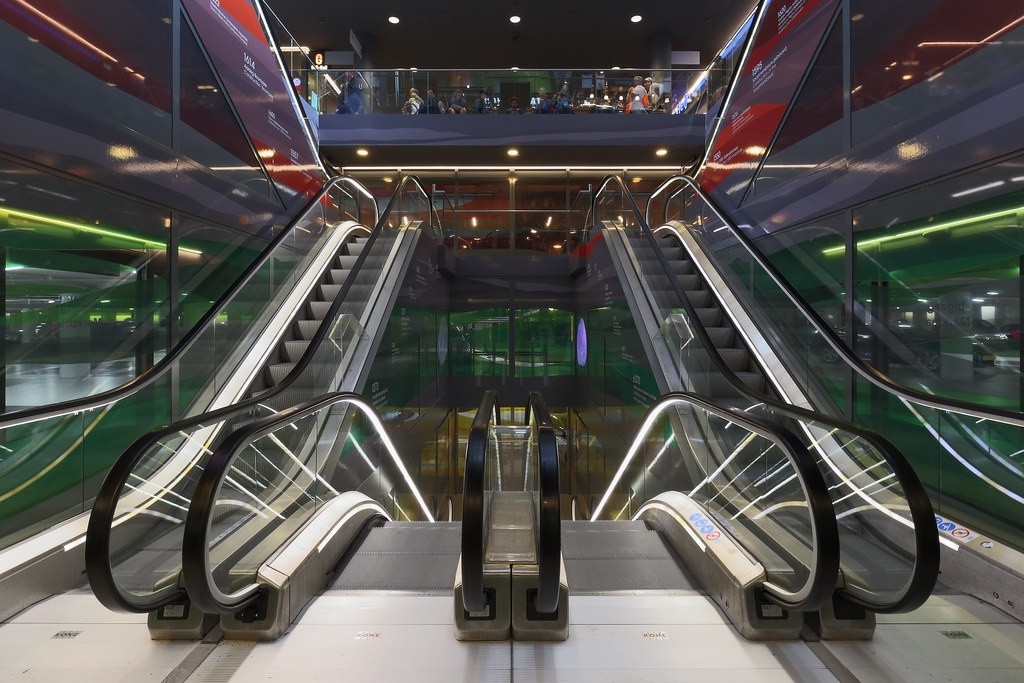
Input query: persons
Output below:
<box><xmin>536</xmin><ymin>76</ymin><xmax>664</xmax><ymax>115</ymax></box>
<box><xmin>506</xmin><ymin>96</ymin><xmax>523</xmax><ymax>114</ymax></box>
<box><xmin>404</xmin><ymin>88</ymin><xmax>494</xmax><ymax>114</ymax></box>
<box><xmin>337</xmin><ymin>72</ymin><xmax>361</xmax><ymax>114</ymax></box>
